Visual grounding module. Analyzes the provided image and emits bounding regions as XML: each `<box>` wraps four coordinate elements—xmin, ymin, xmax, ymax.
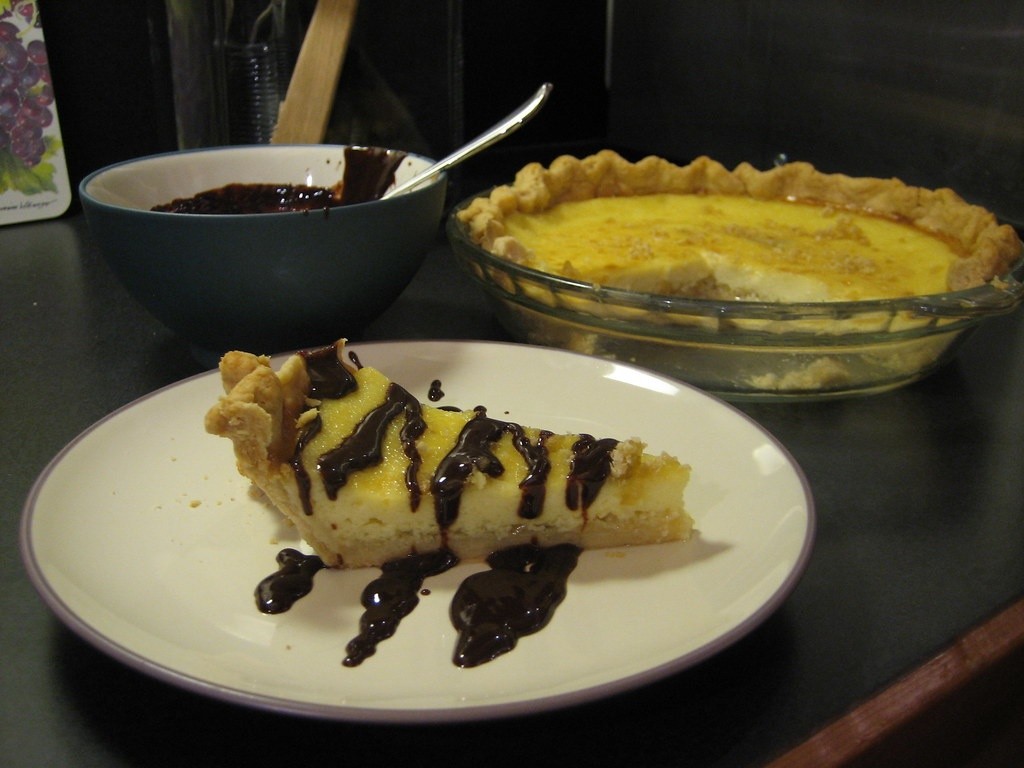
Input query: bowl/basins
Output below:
<box><xmin>442</xmin><ymin>168</ymin><xmax>1024</xmax><ymax>401</ymax></box>
<box><xmin>77</xmin><ymin>143</ymin><xmax>452</xmax><ymax>371</ymax></box>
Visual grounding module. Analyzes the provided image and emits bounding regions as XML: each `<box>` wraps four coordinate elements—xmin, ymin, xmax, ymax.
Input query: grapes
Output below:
<box><xmin>0</xmin><ymin>0</ymin><xmax>54</xmax><ymax>168</ymax></box>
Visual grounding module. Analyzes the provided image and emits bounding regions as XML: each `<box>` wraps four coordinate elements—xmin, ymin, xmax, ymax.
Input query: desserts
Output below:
<box><xmin>204</xmin><ymin>336</ymin><xmax>690</xmax><ymax>565</ymax></box>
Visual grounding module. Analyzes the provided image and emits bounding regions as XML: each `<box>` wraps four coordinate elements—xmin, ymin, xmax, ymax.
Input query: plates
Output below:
<box><xmin>18</xmin><ymin>342</ymin><xmax>818</xmax><ymax>723</ymax></box>
